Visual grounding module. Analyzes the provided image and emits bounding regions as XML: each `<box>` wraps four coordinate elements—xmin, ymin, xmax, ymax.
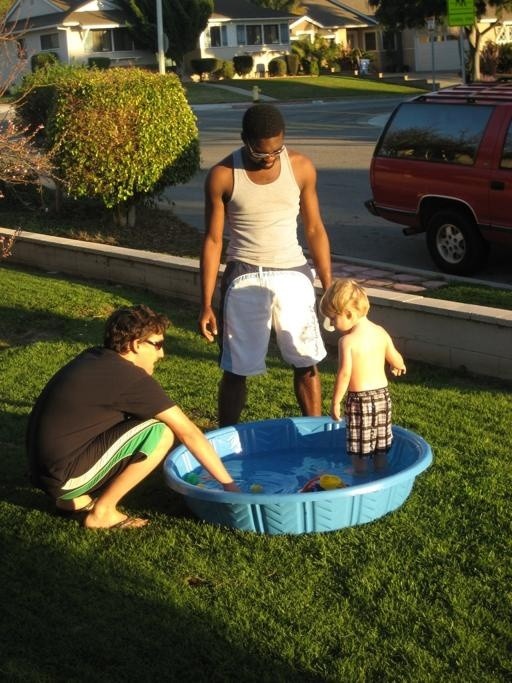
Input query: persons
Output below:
<box><xmin>197</xmin><ymin>102</ymin><xmax>332</xmax><ymax>428</ymax></box>
<box><xmin>25</xmin><ymin>303</ymin><xmax>241</xmax><ymax>530</ymax></box>
<box><xmin>319</xmin><ymin>276</ymin><xmax>406</xmax><ymax>475</ymax></box>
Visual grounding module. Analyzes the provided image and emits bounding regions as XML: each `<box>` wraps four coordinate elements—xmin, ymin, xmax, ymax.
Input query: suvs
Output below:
<box><xmin>363</xmin><ymin>86</ymin><xmax>511</xmax><ymax>269</ymax></box>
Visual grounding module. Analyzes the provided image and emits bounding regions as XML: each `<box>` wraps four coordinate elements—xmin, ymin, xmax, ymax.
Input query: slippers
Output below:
<box><xmin>83</xmin><ymin>515</ymin><xmax>151</xmax><ymax>529</ymax></box>
<box><xmin>54</xmin><ymin>501</ymin><xmax>96</xmax><ymax>514</ymax></box>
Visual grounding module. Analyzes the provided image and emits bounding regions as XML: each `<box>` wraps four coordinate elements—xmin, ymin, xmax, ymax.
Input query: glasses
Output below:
<box><xmin>245</xmin><ymin>144</ymin><xmax>284</xmax><ymax>158</ymax></box>
<box><xmin>145</xmin><ymin>340</ymin><xmax>163</xmax><ymax>349</ymax></box>
